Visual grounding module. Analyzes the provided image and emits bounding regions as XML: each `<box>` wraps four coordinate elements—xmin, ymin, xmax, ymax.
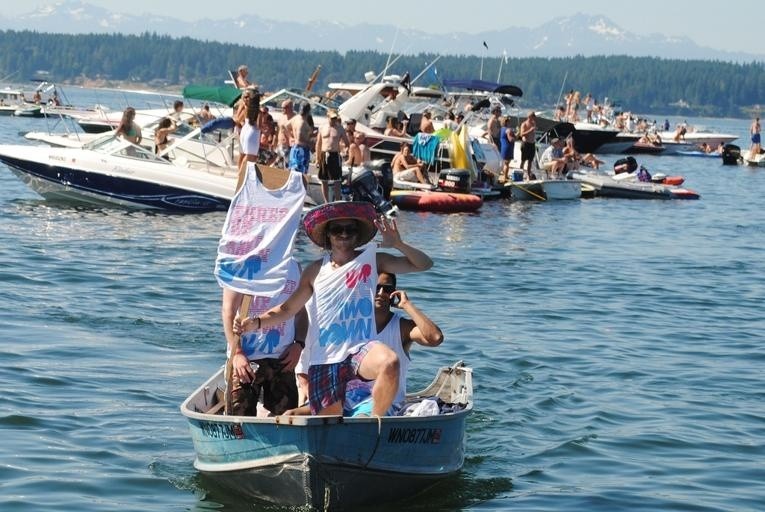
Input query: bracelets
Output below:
<box><xmin>293</xmin><ymin>339</ymin><xmax>306</xmax><ymax>349</ymax></box>
<box><xmin>233</xmin><ymin>348</ymin><xmax>243</xmax><ymax>355</ymax></box>
<box><xmin>257</xmin><ymin>317</ymin><xmax>261</xmax><ymax>330</ymax></box>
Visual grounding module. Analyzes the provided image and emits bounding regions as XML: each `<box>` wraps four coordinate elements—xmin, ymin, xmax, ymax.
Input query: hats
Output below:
<box><xmin>301</xmin><ymin>199</ymin><xmax>380</xmax><ymax>250</ymax></box>
<box><xmin>327</xmin><ymin>112</ymin><xmax>338</xmax><ymax>120</ymax></box>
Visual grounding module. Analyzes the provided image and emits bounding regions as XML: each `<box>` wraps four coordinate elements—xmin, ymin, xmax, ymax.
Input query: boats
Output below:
<box><xmin>181</xmin><ymin>356</ymin><xmax>476</xmax><ymax>512</ymax></box>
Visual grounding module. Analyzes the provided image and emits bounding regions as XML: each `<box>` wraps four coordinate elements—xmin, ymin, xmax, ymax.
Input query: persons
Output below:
<box><xmin>0</xmin><ymin>99</ymin><xmax>10</xmax><ymax>106</ymax></box>
<box><xmin>232</xmin><ymin>67</ymin><xmax>539</xmax><ymax>192</ymax></box>
<box><xmin>114</xmin><ymin>100</ymin><xmax>215</xmax><ymax>162</ymax></box>
<box><xmin>222</xmin><ymin>259</ymin><xmax>309</xmax><ymax>417</ymax></box>
<box><xmin>295</xmin><ymin>270</ymin><xmax>444</xmax><ymax>419</ymax></box>
<box><xmin>231</xmin><ymin>201</ymin><xmax>434</xmax><ymax>418</ymax></box>
<box><xmin>20</xmin><ymin>91</ymin><xmax>59</xmax><ymax>107</ymax></box>
<box><xmin>536</xmin><ymin>86</ymin><xmax>763</xmax><ymax>190</ymax></box>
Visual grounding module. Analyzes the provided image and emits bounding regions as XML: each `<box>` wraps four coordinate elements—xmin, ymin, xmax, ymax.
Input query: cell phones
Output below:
<box><xmin>388</xmin><ymin>292</ymin><xmax>397</xmax><ymax>309</ymax></box>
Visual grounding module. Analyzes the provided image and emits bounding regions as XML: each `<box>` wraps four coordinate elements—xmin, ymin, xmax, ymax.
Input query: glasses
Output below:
<box><xmin>327</xmin><ymin>223</ymin><xmax>361</xmax><ymax>238</ymax></box>
<box><xmin>375</xmin><ymin>283</ymin><xmax>396</xmax><ymax>295</ymax></box>
